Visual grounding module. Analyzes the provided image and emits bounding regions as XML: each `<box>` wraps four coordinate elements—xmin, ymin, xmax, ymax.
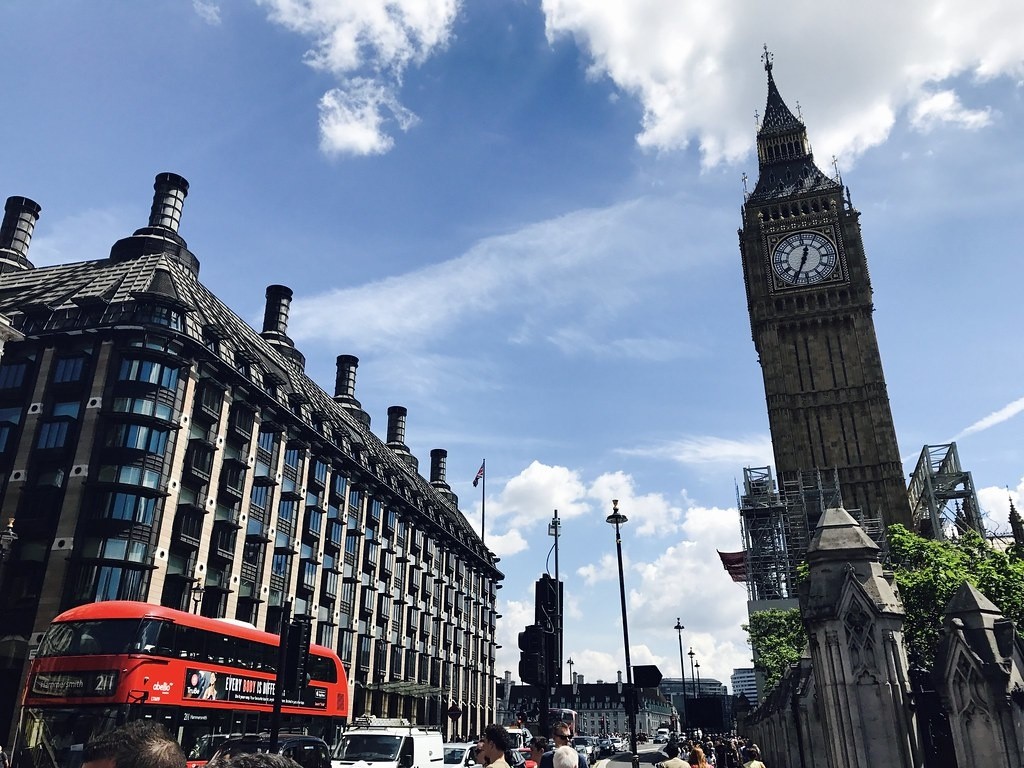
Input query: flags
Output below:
<box><xmin>473</xmin><ymin>463</ymin><xmax>483</xmax><ymax>487</ymax></box>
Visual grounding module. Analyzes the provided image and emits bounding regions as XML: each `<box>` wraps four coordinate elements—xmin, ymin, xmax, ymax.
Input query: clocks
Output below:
<box><xmin>771</xmin><ymin>230</ymin><xmax>839</xmax><ymax>286</ymax></box>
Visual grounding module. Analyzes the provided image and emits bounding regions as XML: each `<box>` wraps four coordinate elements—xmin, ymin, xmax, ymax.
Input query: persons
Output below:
<box><xmin>80</xmin><ymin>715</ymin><xmax>774</xmax><ymax>768</ymax></box>
<box><xmin>201</xmin><ymin>672</ymin><xmax>217</xmax><ymax>701</ymax></box>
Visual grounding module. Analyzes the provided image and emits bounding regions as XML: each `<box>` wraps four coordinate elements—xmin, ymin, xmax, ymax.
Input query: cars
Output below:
<box><xmin>444</xmin><ymin>742</ymin><xmax>479</xmax><ymax>768</ymax></box>
<box><xmin>505</xmin><ymin>726</ymin><xmax>622</xmax><ymax>768</ymax></box>
<box><xmin>653</xmin><ymin>734</ymin><xmax>670</xmax><ymax>744</ymax></box>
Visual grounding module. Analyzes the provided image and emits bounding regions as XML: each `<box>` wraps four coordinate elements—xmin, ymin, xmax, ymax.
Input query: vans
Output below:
<box><xmin>330</xmin><ymin>715</ymin><xmax>445</xmax><ymax>768</ymax></box>
<box><xmin>658</xmin><ymin>728</ymin><xmax>669</xmax><ymax>736</ymax></box>
<box><xmin>201</xmin><ymin>734</ymin><xmax>331</xmax><ymax>768</ymax></box>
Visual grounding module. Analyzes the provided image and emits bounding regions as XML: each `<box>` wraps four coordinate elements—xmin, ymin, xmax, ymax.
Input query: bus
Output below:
<box><xmin>7</xmin><ymin>599</ymin><xmax>350</xmax><ymax>768</ymax></box>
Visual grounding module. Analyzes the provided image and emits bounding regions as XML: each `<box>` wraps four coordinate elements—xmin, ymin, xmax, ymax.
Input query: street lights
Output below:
<box><xmin>605</xmin><ymin>499</ymin><xmax>641</xmax><ymax>768</ymax></box>
<box><xmin>687</xmin><ymin>647</ymin><xmax>700</xmax><ymax>740</ymax></box>
<box><xmin>694</xmin><ymin>661</ymin><xmax>700</xmax><ymax>695</ymax></box>
<box><xmin>674</xmin><ymin>617</ymin><xmax>688</xmax><ymax>738</ymax></box>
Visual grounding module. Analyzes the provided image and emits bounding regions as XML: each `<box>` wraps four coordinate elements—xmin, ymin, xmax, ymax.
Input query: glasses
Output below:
<box><xmin>554</xmin><ymin>734</ymin><xmax>574</xmax><ymax>739</ymax></box>
<box><xmin>475</xmin><ymin>747</ymin><xmax>484</xmax><ymax>754</ymax></box>
